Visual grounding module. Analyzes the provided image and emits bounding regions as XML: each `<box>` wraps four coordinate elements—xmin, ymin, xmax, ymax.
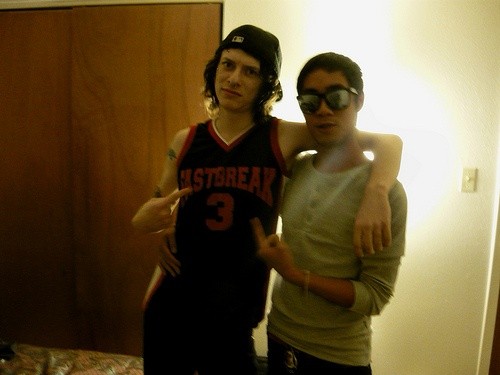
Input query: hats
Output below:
<box><xmin>218</xmin><ymin>24</ymin><xmax>283</xmax><ymax>74</ymax></box>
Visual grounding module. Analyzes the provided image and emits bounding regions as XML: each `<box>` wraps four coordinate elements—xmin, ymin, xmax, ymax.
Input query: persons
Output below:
<box><xmin>149</xmin><ymin>52</ymin><xmax>407</xmax><ymax>375</ymax></box>
<box><xmin>131</xmin><ymin>22</ymin><xmax>405</xmax><ymax>375</ymax></box>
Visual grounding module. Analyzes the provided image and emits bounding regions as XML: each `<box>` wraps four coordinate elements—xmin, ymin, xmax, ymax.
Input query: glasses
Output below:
<box><xmin>292</xmin><ymin>87</ymin><xmax>358</xmax><ymax>116</ymax></box>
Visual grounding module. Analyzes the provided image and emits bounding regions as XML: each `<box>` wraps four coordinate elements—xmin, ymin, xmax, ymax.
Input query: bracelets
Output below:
<box><xmin>301</xmin><ymin>269</ymin><xmax>311</xmax><ymax>289</ymax></box>
